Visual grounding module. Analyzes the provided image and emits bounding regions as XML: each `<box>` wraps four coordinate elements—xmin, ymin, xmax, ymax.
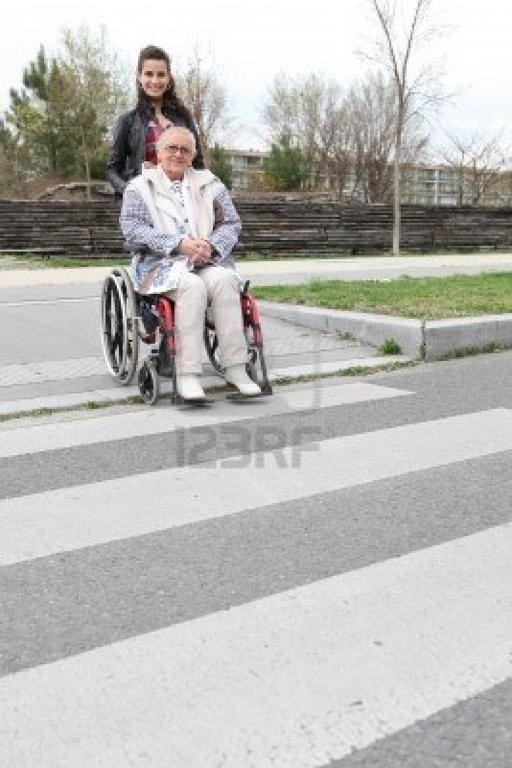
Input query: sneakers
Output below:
<box><xmin>177</xmin><ymin>374</ymin><xmax>205</xmax><ymax>400</ymax></box>
<box><xmin>225</xmin><ymin>368</ymin><xmax>262</xmax><ymax>396</ymax></box>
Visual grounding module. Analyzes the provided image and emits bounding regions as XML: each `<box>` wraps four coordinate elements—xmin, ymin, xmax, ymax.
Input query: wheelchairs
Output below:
<box><xmin>99</xmin><ymin>264</ymin><xmax>272</xmax><ymax>406</ymax></box>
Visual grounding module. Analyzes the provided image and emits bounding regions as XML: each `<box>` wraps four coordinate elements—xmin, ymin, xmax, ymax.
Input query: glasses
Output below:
<box><xmin>164</xmin><ymin>144</ymin><xmax>192</xmax><ymax>156</ymax></box>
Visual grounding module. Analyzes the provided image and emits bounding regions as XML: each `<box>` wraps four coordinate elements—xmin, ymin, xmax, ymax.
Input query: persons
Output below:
<box><xmin>119</xmin><ymin>126</ymin><xmax>261</xmax><ymax>400</ymax></box>
<box><xmin>105</xmin><ymin>46</ymin><xmax>206</xmax><ymax>376</ymax></box>
